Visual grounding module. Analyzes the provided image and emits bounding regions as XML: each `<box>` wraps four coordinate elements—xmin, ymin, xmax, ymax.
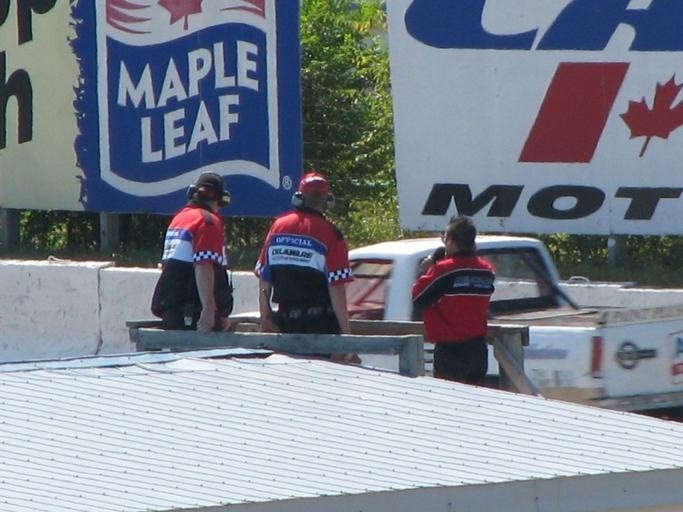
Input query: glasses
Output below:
<box><xmin>442</xmin><ymin>231</ymin><xmax>450</xmax><ymax>243</ymax></box>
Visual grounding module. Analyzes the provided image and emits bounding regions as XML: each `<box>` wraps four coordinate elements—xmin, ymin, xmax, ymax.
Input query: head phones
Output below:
<box><xmin>291</xmin><ymin>177</ymin><xmax>335</xmax><ymax>209</ymax></box>
<box><xmin>186</xmin><ymin>171</ymin><xmax>230</xmax><ymax>207</ymax></box>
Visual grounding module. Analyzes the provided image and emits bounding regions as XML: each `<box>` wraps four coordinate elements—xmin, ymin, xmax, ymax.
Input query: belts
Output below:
<box><xmin>279</xmin><ymin>306</ymin><xmax>332</xmax><ymax>318</ymax></box>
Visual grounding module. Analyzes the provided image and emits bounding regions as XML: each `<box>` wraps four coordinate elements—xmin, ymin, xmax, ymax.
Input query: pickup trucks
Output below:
<box><xmin>226</xmin><ymin>235</ymin><xmax>682</xmax><ymax>423</ymax></box>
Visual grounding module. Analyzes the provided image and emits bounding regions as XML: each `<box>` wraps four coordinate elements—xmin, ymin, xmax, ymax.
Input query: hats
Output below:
<box><xmin>194</xmin><ymin>173</ymin><xmax>223</xmax><ymax>195</ymax></box>
<box><xmin>299</xmin><ymin>176</ymin><xmax>328</xmax><ymax>194</ymax></box>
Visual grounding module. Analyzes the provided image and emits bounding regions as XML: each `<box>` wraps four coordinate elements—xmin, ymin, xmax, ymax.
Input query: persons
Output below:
<box><xmin>252</xmin><ymin>171</ymin><xmax>360</xmax><ymax>366</ymax></box>
<box><xmin>409</xmin><ymin>214</ymin><xmax>495</xmax><ymax>386</ymax></box>
<box><xmin>148</xmin><ymin>170</ymin><xmax>235</xmax><ymax>333</ymax></box>
